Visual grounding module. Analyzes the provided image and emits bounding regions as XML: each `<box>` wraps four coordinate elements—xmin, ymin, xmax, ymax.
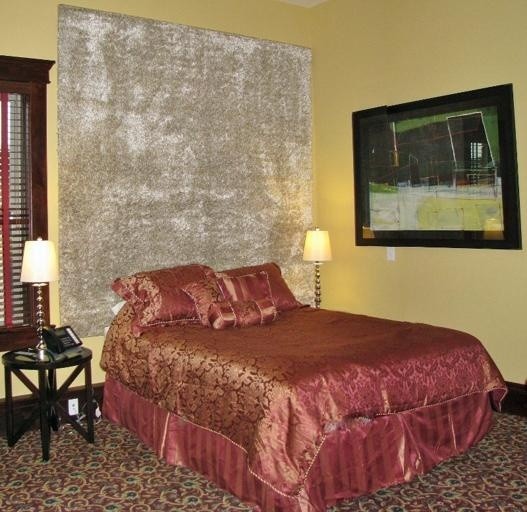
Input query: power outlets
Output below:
<box><xmin>68</xmin><ymin>398</ymin><xmax>79</xmax><ymax>416</ymax></box>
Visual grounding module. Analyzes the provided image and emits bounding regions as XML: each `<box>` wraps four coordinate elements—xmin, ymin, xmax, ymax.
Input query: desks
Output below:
<box><xmin>2</xmin><ymin>346</ymin><xmax>95</xmax><ymax>464</ymax></box>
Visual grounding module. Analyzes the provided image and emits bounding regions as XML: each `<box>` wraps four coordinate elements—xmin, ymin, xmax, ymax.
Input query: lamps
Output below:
<box><xmin>303</xmin><ymin>226</ymin><xmax>334</xmax><ymax>311</ymax></box>
<box><xmin>17</xmin><ymin>234</ymin><xmax>61</xmax><ymax>359</ymax></box>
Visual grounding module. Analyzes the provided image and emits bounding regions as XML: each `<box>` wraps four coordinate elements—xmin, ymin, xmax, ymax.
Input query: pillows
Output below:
<box><xmin>206</xmin><ymin>300</ymin><xmax>280</xmax><ymax>331</ymax></box>
<box><xmin>181</xmin><ymin>262</ymin><xmax>311</xmax><ymax>330</ymax></box>
<box><xmin>110</xmin><ymin>265</ymin><xmax>216</xmax><ymax>336</ymax></box>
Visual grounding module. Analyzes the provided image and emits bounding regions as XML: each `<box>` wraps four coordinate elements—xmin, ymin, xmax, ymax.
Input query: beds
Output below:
<box><xmin>98</xmin><ymin>262</ymin><xmax>511</xmax><ymax>512</ymax></box>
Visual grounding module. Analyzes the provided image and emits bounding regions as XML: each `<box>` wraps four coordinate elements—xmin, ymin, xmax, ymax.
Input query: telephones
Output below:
<box><xmin>42</xmin><ymin>326</ymin><xmax>83</xmax><ymax>354</ymax></box>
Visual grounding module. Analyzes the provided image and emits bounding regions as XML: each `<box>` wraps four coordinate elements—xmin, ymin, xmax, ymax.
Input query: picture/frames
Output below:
<box><xmin>349</xmin><ymin>80</ymin><xmax>524</xmax><ymax>252</ymax></box>
